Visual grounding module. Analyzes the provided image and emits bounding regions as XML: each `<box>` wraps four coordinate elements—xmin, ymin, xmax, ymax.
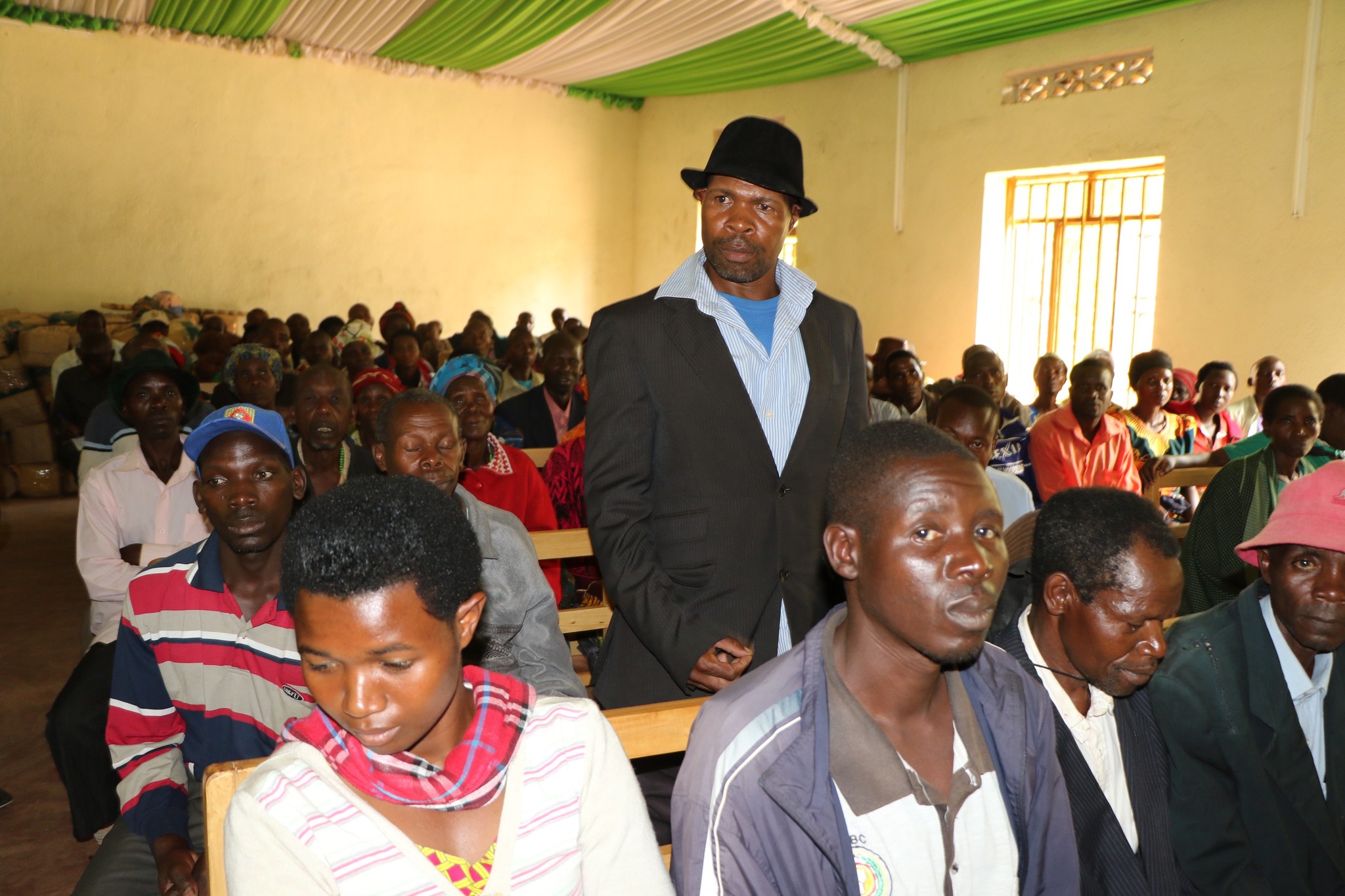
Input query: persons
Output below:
<box><xmin>45</xmin><ymin>294</ymin><xmax>1345</xmax><ymax>653</ymax></box>
<box><xmin>992</xmin><ymin>485</ymin><xmax>1185</xmax><ymax>896</ymax></box>
<box><xmin>667</xmin><ymin>419</ymin><xmax>1083</xmax><ymax>896</ymax></box>
<box><xmin>222</xmin><ymin>472</ymin><xmax>680</xmax><ymax>896</ymax></box>
<box><xmin>368</xmin><ymin>387</ymin><xmax>594</xmax><ymax>703</ymax></box>
<box><xmin>580</xmin><ymin>116</ymin><xmax>876</xmax><ymax>846</ymax></box>
<box><xmin>66</xmin><ymin>401</ymin><xmax>310</xmax><ymax>896</ymax></box>
<box><xmin>1146</xmin><ymin>457</ymin><xmax>1345</xmax><ymax>896</ymax></box>
<box><xmin>41</xmin><ymin>346</ymin><xmax>212</xmax><ymax>846</ymax></box>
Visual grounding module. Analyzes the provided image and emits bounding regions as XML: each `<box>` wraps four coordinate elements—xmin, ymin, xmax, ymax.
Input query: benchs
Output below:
<box><xmin>1145</xmin><ymin>464</ymin><xmax>1228</xmax><ymax>543</ymax></box>
<box><xmin>204</xmin><ymin>692</ymin><xmax>731</xmax><ymax>896</ymax></box>
<box><xmin>514</xmin><ymin>528</ymin><xmax>613</xmax><ymax>691</ymax></box>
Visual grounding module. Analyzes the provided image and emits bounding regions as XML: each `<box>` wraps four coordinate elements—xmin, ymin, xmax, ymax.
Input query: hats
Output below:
<box><xmin>681</xmin><ymin>116</ymin><xmax>818</xmax><ymax>219</ymax></box>
<box><xmin>181</xmin><ymin>402</ymin><xmax>296</xmax><ymax>484</ymax></box>
<box><xmin>1233</xmin><ymin>458</ymin><xmax>1345</xmax><ymax>568</ymax></box>
<box><xmin>865</xmin><ymin>336</ymin><xmax>928</xmax><ymax>367</ymax></box>
<box><xmin>107</xmin><ymin>347</ymin><xmax>201</xmax><ymax>418</ymax></box>
<box><xmin>140</xmin><ymin>309</ymin><xmax>170</xmax><ymax>329</ymax></box>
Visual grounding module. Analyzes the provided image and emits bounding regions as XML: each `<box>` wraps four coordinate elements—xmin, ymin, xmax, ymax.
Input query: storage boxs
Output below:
<box><xmin>0</xmin><ymin>306</ymin><xmax>238</xmax><ymax>495</ymax></box>
